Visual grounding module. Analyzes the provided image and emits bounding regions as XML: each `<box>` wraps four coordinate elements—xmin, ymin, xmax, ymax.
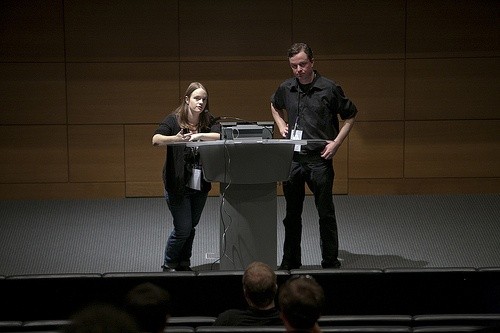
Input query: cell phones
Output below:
<box><xmin>183</xmin><ymin>128</ymin><xmax>189</xmax><ymax>135</ymax></box>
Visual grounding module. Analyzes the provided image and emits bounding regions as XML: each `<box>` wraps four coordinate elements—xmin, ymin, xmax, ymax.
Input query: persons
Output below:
<box><xmin>278</xmin><ymin>275</ymin><xmax>326</xmax><ymax>333</ymax></box>
<box><xmin>122</xmin><ymin>283</ymin><xmax>172</xmax><ymax>333</ymax></box>
<box><xmin>211</xmin><ymin>261</ymin><xmax>284</xmax><ymax>327</ymax></box>
<box><xmin>151</xmin><ymin>81</ymin><xmax>221</xmax><ymax>272</ymax></box>
<box><xmin>270</xmin><ymin>42</ymin><xmax>358</xmax><ymax>271</ymax></box>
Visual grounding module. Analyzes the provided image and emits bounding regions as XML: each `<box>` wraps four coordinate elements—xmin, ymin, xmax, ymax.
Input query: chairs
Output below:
<box><xmin>0</xmin><ymin>265</ymin><xmax>500</xmax><ymax>333</ymax></box>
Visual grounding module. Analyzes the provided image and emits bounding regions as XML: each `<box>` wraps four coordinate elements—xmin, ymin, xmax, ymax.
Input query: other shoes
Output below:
<box><xmin>279</xmin><ymin>261</ymin><xmax>300</xmax><ymax>269</ymax></box>
<box><xmin>321</xmin><ymin>258</ymin><xmax>341</xmax><ymax>268</ymax></box>
<box><xmin>161</xmin><ymin>266</ymin><xmax>193</xmax><ymax>272</ymax></box>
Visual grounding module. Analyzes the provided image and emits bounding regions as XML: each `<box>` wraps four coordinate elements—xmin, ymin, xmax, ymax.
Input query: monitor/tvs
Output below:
<box><xmin>220</xmin><ymin>120</ymin><xmax>274</xmax><ymax>140</ymax></box>
